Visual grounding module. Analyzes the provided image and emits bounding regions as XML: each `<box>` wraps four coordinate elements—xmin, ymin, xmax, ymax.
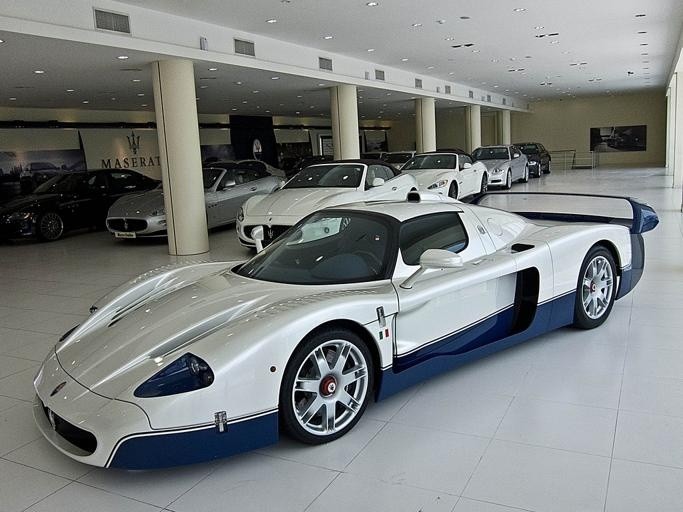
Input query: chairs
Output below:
<box><xmin>335</xmin><ymin>219</ymin><xmax>376</xmax><ymax>259</ymax></box>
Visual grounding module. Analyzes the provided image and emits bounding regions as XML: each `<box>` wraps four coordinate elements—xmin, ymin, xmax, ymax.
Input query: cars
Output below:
<box><xmin>17</xmin><ymin>162</ymin><xmax>60</xmax><ymax>188</ymax></box>
<box><xmin>1</xmin><ymin>168</ymin><xmax>166</xmax><ymax>244</ymax></box>
<box><xmin>607</xmin><ymin>134</ymin><xmax>639</xmax><ymax>148</ymax></box>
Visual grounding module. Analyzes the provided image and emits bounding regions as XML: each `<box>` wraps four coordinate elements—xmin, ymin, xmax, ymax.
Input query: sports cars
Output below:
<box><xmin>30</xmin><ymin>187</ymin><xmax>660</xmax><ymax>470</ymax></box>
